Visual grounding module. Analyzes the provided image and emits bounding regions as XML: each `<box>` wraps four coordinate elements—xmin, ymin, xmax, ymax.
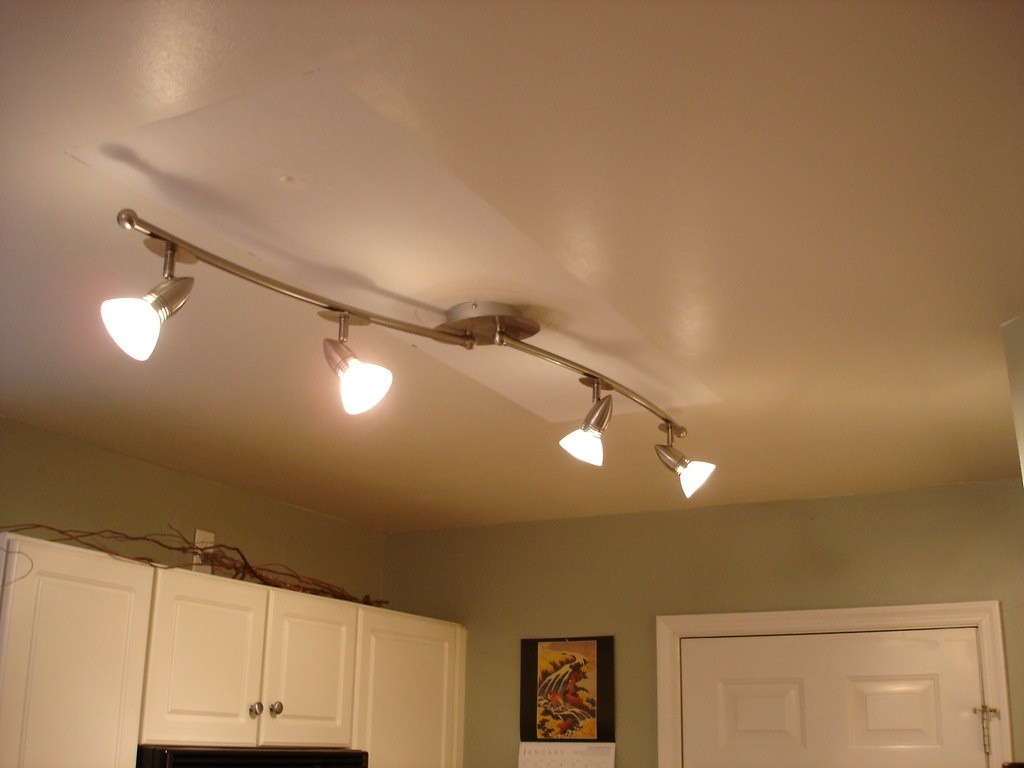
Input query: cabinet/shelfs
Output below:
<box><xmin>0</xmin><ymin>532</ymin><xmax>468</xmax><ymax>768</ymax></box>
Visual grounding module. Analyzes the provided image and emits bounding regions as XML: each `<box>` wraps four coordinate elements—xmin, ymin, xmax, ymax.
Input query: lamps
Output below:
<box><xmin>317</xmin><ymin>309</ymin><xmax>393</xmax><ymax>416</ymax></box>
<box><xmin>655</xmin><ymin>421</ymin><xmax>716</xmax><ymax>499</ymax></box>
<box><xmin>558</xmin><ymin>376</ymin><xmax>614</xmax><ymax>467</ymax></box>
<box><xmin>101</xmin><ymin>236</ymin><xmax>198</xmax><ymax>361</ymax></box>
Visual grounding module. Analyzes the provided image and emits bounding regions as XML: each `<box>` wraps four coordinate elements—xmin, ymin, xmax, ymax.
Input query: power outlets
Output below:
<box><xmin>192</xmin><ymin>529</ymin><xmax>215</xmax><ymax>575</ymax></box>
